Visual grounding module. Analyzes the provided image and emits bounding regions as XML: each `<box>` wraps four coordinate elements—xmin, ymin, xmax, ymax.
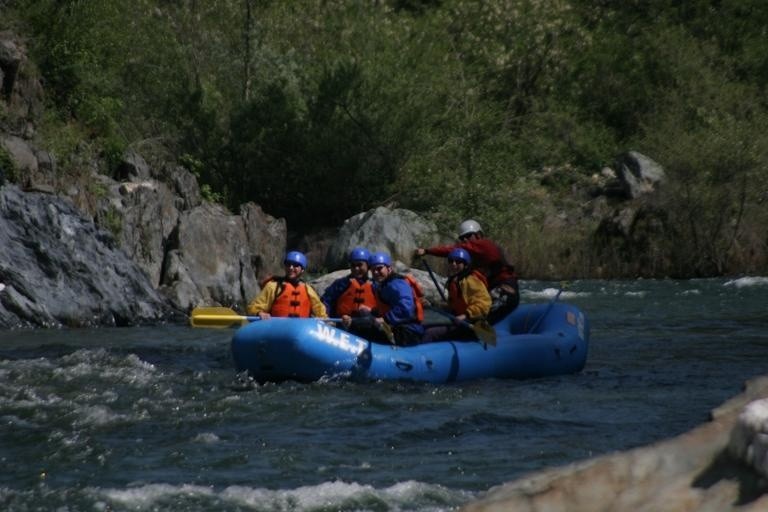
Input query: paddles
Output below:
<box><xmin>190</xmin><ymin>307</ymin><xmax>343</xmax><ymax>329</ymax></box>
<box><xmin>421</xmin><ymin>304</ymin><xmax>496</xmax><ymax>345</ymax></box>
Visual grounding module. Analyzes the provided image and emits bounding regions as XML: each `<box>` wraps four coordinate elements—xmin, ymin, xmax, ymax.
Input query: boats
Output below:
<box><xmin>232</xmin><ymin>299</ymin><xmax>587</xmax><ymax>387</ymax></box>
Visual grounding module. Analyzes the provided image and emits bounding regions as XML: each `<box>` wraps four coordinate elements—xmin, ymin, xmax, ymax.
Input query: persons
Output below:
<box><xmin>421</xmin><ymin>247</ymin><xmax>492</xmax><ymax>343</ymax></box>
<box><xmin>367</xmin><ymin>251</ymin><xmax>426</xmax><ymax>346</ymax></box>
<box><xmin>320</xmin><ymin>247</ymin><xmax>378</xmax><ymax>345</ymax></box>
<box><xmin>415</xmin><ymin>218</ymin><xmax>520</xmax><ymax>327</ymax></box>
<box><xmin>245</xmin><ymin>250</ymin><xmax>336</xmax><ymax>328</ymax></box>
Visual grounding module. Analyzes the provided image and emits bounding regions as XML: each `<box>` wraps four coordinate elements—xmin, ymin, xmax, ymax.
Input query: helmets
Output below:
<box><xmin>447</xmin><ymin>248</ymin><xmax>470</xmax><ymax>263</ymax></box>
<box><xmin>350</xmin><ymin>247</ymin><xmax>368</xmax><ymax>261</ymax></box>
<box><xmin>367</xmin><ymin>251</ymin><xmax>392</xmax><ymax>266</ymax></box>
<box><xmin>458</xmin><ymin>220</ymin><xmax>484</xmax><ymax>237</ymax></box>
<box><xmin>283</xmin><ymin>251</ymin><xmax>308</xmax><ymax>269</ymax></box>
